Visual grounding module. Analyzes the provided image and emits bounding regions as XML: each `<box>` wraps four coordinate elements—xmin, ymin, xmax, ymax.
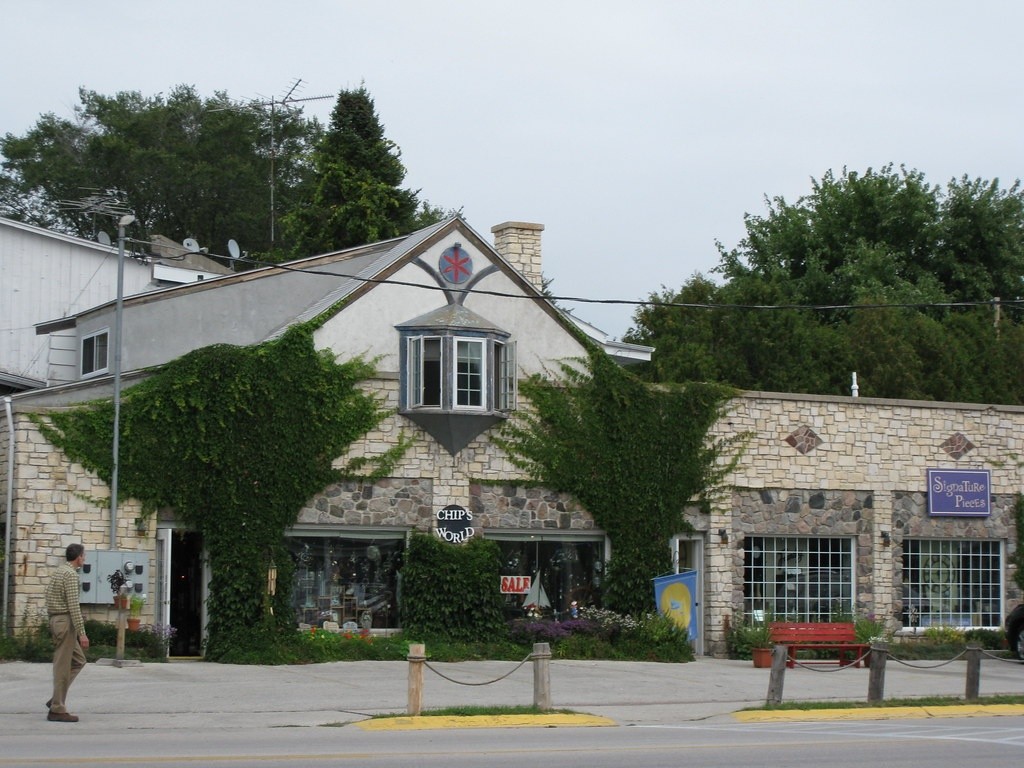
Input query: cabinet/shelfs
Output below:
<box><xmin>300</xmin><ymin>584</ymin><xmax>372</xmax><ymax>628</ymax></box>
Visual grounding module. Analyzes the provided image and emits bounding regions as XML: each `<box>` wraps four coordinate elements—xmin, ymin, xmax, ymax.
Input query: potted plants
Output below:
<box><xmin>107</xmin><ymin>570</ymin><xmax>130</xmax><ymax>609</ymax></box>
<box><xmin>127</xmin><ymin>594</ymin><xmax>145</xmax><ymax>632</ymax></box>
<box><xmin>137</xmin><ymin>522</ymin><xmax>147</xmax><ymax>536</ymax></box>
<box><xmin>733</xmin><ymin>609</ymin><xmax>777</xmax><ymax>668</ymax></box>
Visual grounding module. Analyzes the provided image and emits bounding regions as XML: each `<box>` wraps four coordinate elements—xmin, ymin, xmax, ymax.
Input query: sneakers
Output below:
<box><xmin>45</xmin><ymin>700</ymin><xmax>51</xmax><ymax>707</ymax></box>
<box><xmin>48</xmin><ymin>713</ymin><xmax>78</xmax><ymax>721</ymax></box>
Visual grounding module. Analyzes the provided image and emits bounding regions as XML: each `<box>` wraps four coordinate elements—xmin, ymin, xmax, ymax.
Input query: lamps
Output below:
<box><xmin>881</xmin><ymin>531</ymin><xmax>890</xmax><ymax>547</ymax></box>
<box><xmin>719</xmin><ymin>528</ymin><xmax>728</xmax><ymax>544</ymax></box>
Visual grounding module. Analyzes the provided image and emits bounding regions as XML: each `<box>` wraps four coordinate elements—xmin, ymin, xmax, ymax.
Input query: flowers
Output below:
<box><xmin>855</xmin><ymin>612</ymin><xmax>891</xmax><ymax>643</ymax></box>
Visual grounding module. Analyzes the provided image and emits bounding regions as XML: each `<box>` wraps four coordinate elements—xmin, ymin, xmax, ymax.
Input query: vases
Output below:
<box><xmin>863</xmin><ymin>649</ymin><xmax>871</xmax><ymax>668</ymax></box>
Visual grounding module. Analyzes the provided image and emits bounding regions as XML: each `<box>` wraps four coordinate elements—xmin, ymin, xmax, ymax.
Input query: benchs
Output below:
<box><xmin>768</xmin><ymin>621</ymin><xmax>870</xmax><ymax>667</ymax></box>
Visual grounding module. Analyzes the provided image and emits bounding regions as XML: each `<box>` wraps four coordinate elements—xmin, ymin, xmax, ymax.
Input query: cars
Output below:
<box><xmin>1005</xmin><ymin>604</ymin><xmax>1024</xmax><ymax>660</ymax></box>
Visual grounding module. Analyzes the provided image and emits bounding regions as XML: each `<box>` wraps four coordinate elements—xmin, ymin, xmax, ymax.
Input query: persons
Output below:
<box><xmin>44</xmin><ymin>543</ymin><xmax>90</xmax><ymax>723</ymax></box>
